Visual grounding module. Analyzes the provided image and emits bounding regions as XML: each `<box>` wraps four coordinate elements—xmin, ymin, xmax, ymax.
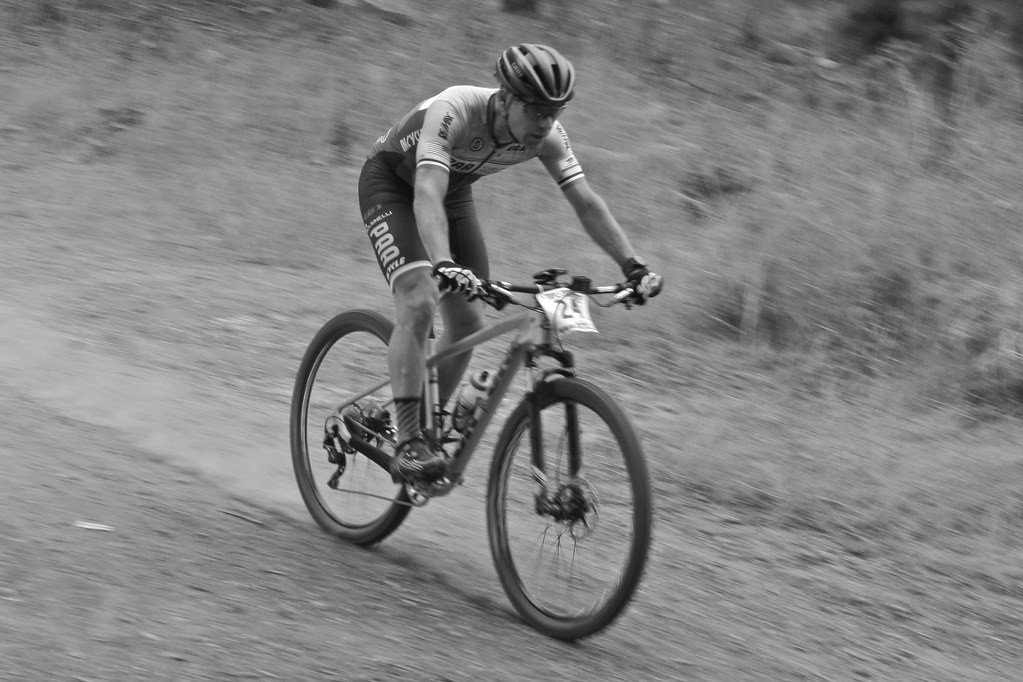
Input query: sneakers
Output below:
<box><xmin>389</xmin><ymin>443</ymin><xmax>447</xmax><ymax>484</ymax></box>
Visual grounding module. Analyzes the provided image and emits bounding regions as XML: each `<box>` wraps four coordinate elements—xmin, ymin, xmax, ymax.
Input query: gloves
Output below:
<box><xmin>431</xmin><ymin>261</ymin><xmax>488</xmax><ymax>302</ymax></box>
<box><xmin>628</xmin><ymin>269</ymin><xmax>664</xmax><ymax>300</ymax></box>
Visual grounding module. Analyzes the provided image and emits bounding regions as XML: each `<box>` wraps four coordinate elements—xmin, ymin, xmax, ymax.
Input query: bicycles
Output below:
<box><xmin>286</xmin><ymin>263</ymin><xmax>666</xmax><ymax>640</ymax></box>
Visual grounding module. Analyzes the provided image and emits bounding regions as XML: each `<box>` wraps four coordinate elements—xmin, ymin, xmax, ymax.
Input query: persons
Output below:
<box><xmin>357</xmin><ymin>43</ymin><xmax>664</xmax><ymax>485</ymax></box>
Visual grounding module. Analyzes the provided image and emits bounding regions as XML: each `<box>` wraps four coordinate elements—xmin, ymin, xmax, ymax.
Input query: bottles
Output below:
<box><xmin>453</xmin><ymin>369</ymin><xmax>490</xmax><ymax>430</ymax></box>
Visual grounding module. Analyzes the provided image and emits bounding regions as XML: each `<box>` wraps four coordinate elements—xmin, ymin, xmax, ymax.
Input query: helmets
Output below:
<box><xmin>492</xmin><ymin>43</ymin><xmax>576</xmax><ymax>107</ymax></box>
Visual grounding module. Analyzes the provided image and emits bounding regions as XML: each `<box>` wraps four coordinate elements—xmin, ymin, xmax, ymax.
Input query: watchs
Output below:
<box><xmin>622</xmin><ymin>256</ymin><xmax>647</xmax><ymax>276</ymax></box>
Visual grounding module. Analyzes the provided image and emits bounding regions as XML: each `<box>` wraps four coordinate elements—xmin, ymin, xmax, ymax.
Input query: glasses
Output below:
<box><xmin>515</xmin><ymin>97</ymin><xmax>568</xmax><ymax>122</ymax></box>
